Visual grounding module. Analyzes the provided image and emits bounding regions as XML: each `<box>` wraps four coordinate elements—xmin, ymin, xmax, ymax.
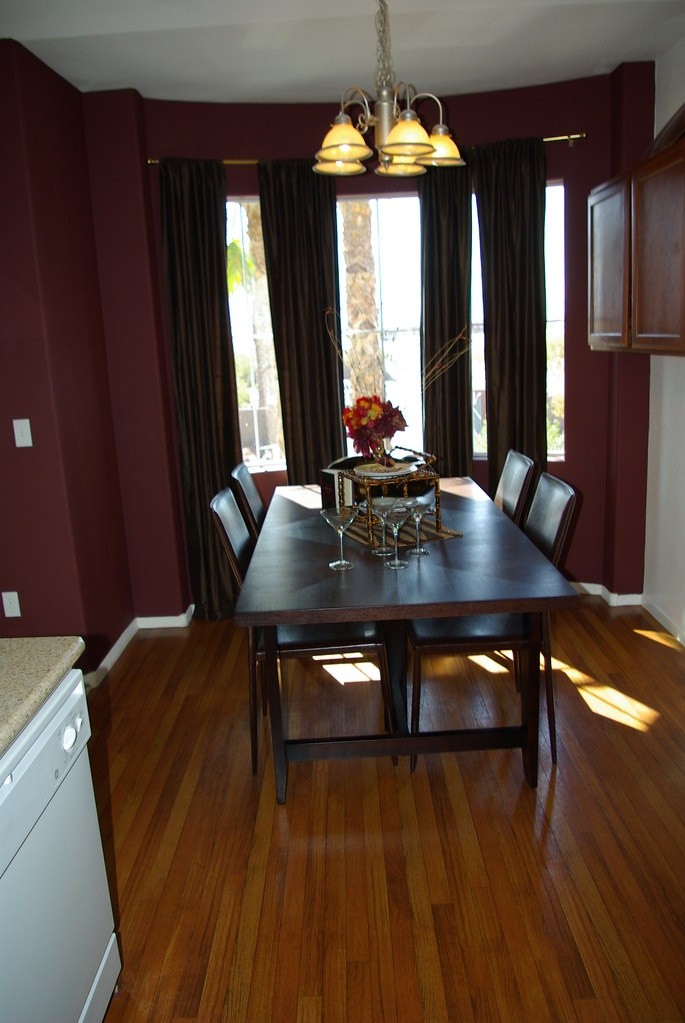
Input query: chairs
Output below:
<box><xmin>206</xmin><ymin>448</ymin><xmax>581</xmax><ymax>805</ymax></box>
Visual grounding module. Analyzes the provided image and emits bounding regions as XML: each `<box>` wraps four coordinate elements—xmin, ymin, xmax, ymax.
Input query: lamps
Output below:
<box><xmin>310</xmin><ymin>1</ymin><xmax>467</xmax><ymax>180</ymax></box>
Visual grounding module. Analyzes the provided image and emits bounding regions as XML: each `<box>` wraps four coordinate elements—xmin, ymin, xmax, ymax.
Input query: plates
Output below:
<box><xmin>353</xmin><ymin>463</ymin><xmax>417</xmax><ymax>480</ymax></box>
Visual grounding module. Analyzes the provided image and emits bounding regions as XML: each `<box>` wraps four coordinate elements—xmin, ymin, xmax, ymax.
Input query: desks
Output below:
<box><xmin>233</xmin><ymin>477</ymin><xmax>578</xmax><ymax>805</ymax></box>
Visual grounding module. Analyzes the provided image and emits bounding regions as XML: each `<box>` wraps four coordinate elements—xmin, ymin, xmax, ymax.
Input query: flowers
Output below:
<box><xmin>342</xmin><ymin>395</ymin><xmax>406</xmax><ymax>469</ymax></box>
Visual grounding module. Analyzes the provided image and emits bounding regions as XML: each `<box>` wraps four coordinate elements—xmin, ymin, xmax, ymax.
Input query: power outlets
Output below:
<box><xmin>2</xmin><ymin>591</ymin><xmax>22</xmax><ymax>618</ymax></box>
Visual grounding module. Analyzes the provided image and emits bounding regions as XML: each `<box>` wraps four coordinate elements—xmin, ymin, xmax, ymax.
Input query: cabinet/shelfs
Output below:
<box><xmin>583</xmin><ymin>153</ymin><xmax>684</xmax><ymax>354</ymax></box>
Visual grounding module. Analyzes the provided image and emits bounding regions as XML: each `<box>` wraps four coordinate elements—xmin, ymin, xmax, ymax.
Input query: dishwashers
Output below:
<box><xmin>0</xmin><ymin>667</ymin><xmax>123</xmax><ymax>1023</ymax></box>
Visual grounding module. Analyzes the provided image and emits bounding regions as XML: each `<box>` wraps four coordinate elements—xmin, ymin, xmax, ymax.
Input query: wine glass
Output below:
<box><xmin>365</xmin><ymin>497</ymin><xmax>399</xmax><ymax>556</ymax></box>
<box><xmin>320</xmin><ymin>507</ymin><xmax>359</xmax><ymax>571</ymax></box>
<box><xmin>371</xmin><ymin>500</ymin><xmax>418</xmax><ymax>571</ymax></box>
<box><xmin>399</xmin><ymin>496</ymin><xmax>435</xmax><ymax>558</ymax></box>
